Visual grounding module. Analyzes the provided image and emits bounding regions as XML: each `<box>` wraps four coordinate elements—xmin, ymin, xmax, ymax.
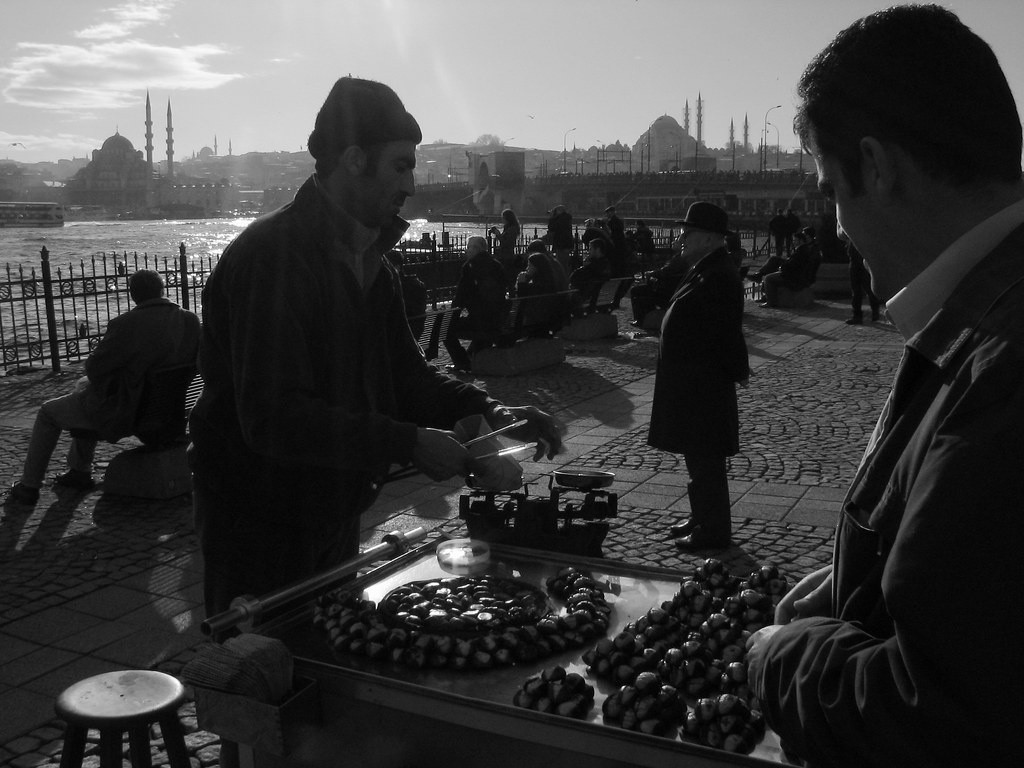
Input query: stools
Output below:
<box><xmin>55</xmin><ymin>669</ymin><xmax>191</xmax><ymax>768</ymax></box>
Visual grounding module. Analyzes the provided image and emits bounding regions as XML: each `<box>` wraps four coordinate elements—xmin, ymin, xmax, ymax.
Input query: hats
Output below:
<box><xmin>604</xmin><ymin>207</ymin><xmax>616</xmax><ymax>212</ymax></box>
<box><xmin>307</xmin><ymin>77</ymin><xmax>423</xmax><ymax>161</ymax></box>
<box><xmin>674</xmin><ymin>201</ymin><xmax>736</xmax><ymax>237</ymax></box>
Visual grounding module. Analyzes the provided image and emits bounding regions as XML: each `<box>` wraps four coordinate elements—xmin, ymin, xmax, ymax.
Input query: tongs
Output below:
<box><xmin>371</xmin><ymin>418</ymin><xmax>537</xmax><ymax>487</ymax></box>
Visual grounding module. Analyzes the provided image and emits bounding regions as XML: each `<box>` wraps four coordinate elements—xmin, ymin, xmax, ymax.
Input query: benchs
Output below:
<box><xmin>68</xmin><ymin>358</ymin><xmax>205</xmax><ymax>500</ymax></box>
<box><xmin>772</xmin><ymin>258</ymin><xmax>822</xmax><ymax>307</ymax></box>
<box><xmin>561</xmin><ymin>275</ymin><xmax>634</xmax><ymax>343</ymax></box>
<box><xmin>408</xmin><ymin>307</ymin><xmax>462</xmax><ymax>363</ymax></box>
<box><xmin>455</xmin><ymin>286</ymin><xmax>581</xmax><ymax>376</ymax></box>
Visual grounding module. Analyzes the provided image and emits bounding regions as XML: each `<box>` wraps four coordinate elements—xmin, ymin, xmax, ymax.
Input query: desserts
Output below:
<box><xmin>310</xmin><ymin>556</ymin><xmax>795</xmax><ymax>754</ymax></box>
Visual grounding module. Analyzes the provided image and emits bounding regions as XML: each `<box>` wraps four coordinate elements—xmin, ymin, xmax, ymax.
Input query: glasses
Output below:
<box><xmin>681</xmin><ymin>230</ymin><xmax>711</xmax><ymax>240</ymax></box>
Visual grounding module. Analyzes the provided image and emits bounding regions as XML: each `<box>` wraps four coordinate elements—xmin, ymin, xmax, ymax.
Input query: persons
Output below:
<box><xmin>844</xmin><ymin>240</ymin><xmax>882</xmax><ymax>325</ymax></box>
<box><xmin>8</xmin><ymin>269</ymin><xmax>202</xmax><ymax>500</ymax></box>
<box><xmin>723</xmin><ymin>229</ymin><xmax>747</xmax><ymax>269</ymax></box>
<box><xmin>746</xmin><ymin>208</ymin><xmax>844</xmax><ymax>308</ymax></box>
<box><xmin>629</xmin><ymin>230</ymin><xmax>695</xmax><ymax>327</ymax></box>
<box><xmin>424</xmin><ymin>203</ymin><xmax>654</xmax><ymax>369</ymax></box>
<box><xmin>191</xmin><ymin>79</ymin><xmax>563</xmax><ymax>641</ymax></box>
<box><xmin>645</xmin><ymin>201</ymin><xmax>752</xmax><ymax>553</ymax></box>
<box><xmin>741</xmin><ymin>1</ymin><xmax>1022</xmax><ymax>768</ymax></box>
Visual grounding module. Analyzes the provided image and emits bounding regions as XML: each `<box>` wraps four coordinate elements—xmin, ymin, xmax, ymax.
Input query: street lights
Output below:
<box><xmin>670</xmin><ymin>131</ymin><xmax>681</xmax><ymax>170</ymax></box>
<box><xmin>503</xmin><ymin>137</ymin><xmax>514</xmax><ymax>151</ymax></box>
<box><xmin>690</xmin><ymin>136</ymin><xmax>698</xmax><ymax>170</ymax></box>
<box><xmin>648</xmin><ymin>117</ymin><xmax>662</xmax><ymax>167</ymax></box>
<box><xmin>564</xmin><ymin>128</ymin><xmax>577</xmax><ymax>172</ymax></box>
<box><xmin>764</xmin><ymin>106</ymin><xmax>781</xmax><ymax>173</ymax></box>
<box><xmin>768</xmin><ymin>122</ymin><xmax>779</xmax><ymax>169</ymax></box>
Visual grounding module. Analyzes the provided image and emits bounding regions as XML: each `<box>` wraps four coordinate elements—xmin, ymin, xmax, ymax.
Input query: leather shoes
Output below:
<box><xmin>674</xmin><ymin>534</ymin><xmax>707</xmax><ymax>551</ymax></box>
<box><xmin>671</xmin><ymin>517</ymin><xmax>695</xmax><ymax>536</ymax></box>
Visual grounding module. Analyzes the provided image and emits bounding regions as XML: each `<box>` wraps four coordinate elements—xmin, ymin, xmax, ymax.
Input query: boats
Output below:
<box><xmin>0</xmin><ymin>202</ymin><xmax>64</xmax><ymax>228</ymax></box>
<box><xmin>392</xmin><ymin>223</ymin><xmax>496</xmax><ymax>265</ymax></box>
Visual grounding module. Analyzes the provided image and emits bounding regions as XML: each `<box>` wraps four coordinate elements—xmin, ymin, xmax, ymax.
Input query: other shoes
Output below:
<box><xmin>745</xmin><ymin>272</ymin><xmax>762</xmax><ymax>283</ymax></box>
<box><xmin>12</xmin><ymin>479</ymin><xmax>40</xmax><ymax>500</ymax></box>
<box><xmin>869</xmin><ymin>303</ymin><xmax>880</xmax><ymax>320</ymax></box>
<box><xmin>630</xmin><ymin>320</ymin><xmax>639</xmax><ymax>326</ymax></box>
<box><xmin>55</xmin><ymin>469</ymin><xmax>92</xmax><ymax>487</ymax></box>
<box><xmin>755</xmin><ymin>294</ymin><xmax>766</xmax><ymax>303</ymax></box>
<box><xmin>446</xmin><ymin>364</ymin><xmax>471</xmax><ymax>371</ymax></box>
<box><xmin>759</xmin><ymin>301</ymin><xmax>773</xmax><ymax>308</ymax></box>
<box><xmin>847</xmin><ymin>310</ymin><xmax>863</xmax><ymax>326</ymax></box>
